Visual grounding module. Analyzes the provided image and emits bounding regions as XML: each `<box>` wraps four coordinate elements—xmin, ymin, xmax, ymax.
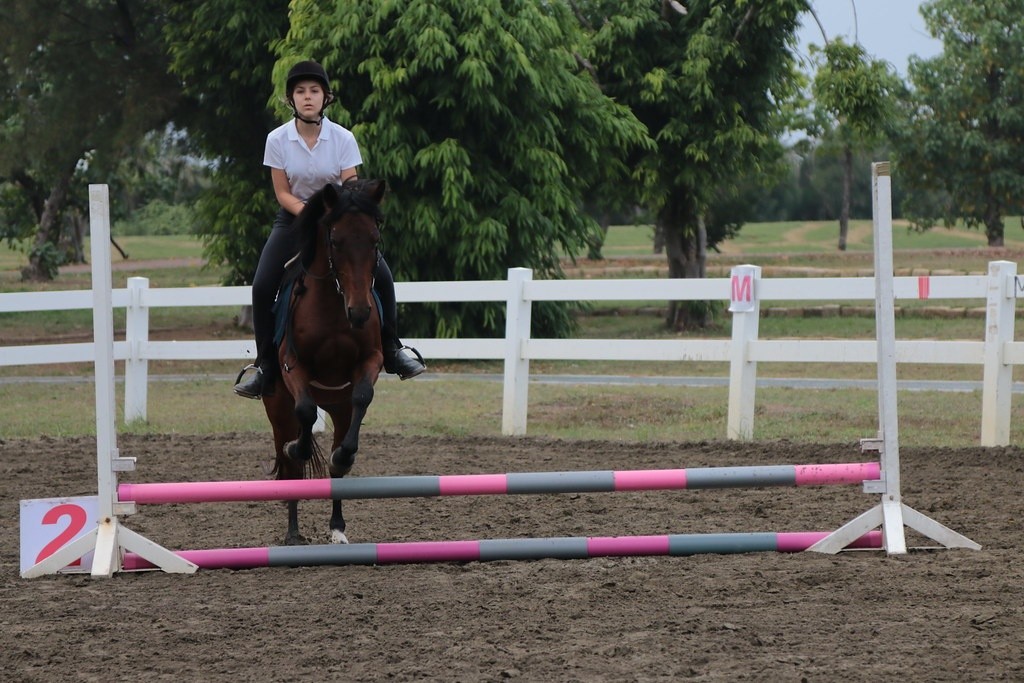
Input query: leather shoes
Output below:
<box><xmin>234</xmin><ymin>370</ymin><xmax>275</xmax><ymax>397</ymax></box>
<box><xmin>383</xmin><ymin>345</ymin><xmax>424</xmax><ymax>379</ymax></box>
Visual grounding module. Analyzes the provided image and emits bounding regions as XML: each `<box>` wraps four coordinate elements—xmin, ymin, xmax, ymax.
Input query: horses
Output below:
<box><xmin>260</xmin><ymin>179</ymin><xmax>382</xmax><ymax>545</ymax></box>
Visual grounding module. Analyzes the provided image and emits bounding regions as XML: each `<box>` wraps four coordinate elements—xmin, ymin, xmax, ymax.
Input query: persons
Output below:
<box><xmin>233</xmin><ymin>61</ymin><xmax>424</xmax><ymax>397</ymax></box>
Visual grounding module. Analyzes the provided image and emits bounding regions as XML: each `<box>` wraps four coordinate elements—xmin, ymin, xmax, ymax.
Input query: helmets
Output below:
<box><xmin>285</xmin><ymin>61</ymin><xmax>330</xmax><ymax>98</ymax></box>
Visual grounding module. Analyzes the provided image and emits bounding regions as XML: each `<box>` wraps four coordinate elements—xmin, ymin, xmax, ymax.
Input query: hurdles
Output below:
<box><xmin>84</xmin><ymin>158</ymin><xmax>983</xmax><ymax>582</ymax></box>
